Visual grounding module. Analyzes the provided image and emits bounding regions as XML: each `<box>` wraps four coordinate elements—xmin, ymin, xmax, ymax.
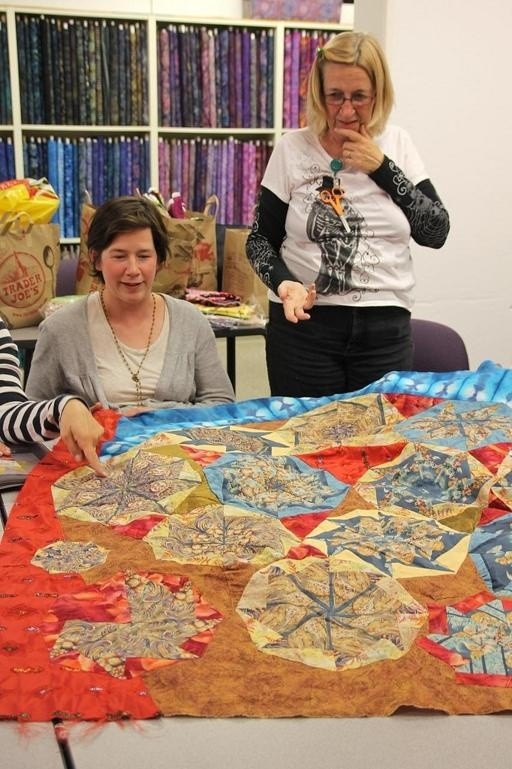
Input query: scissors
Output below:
<box><xmin>320</xmin><ymin>186</ymin><xmax>352</xmax><ymax>233</ymax></box>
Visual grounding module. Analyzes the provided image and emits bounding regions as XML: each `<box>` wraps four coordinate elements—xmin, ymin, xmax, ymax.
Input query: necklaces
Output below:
<box><xmin>96</xmin><ymin>286</ymin><xmax>156</xmax><ymax>407</ymax></box>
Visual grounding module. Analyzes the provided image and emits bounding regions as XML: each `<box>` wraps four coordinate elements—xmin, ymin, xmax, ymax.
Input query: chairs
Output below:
<box><xmin>408</xmin><ymin>309</ymin><xmax>469</xmax><ymax>369</ymax></box>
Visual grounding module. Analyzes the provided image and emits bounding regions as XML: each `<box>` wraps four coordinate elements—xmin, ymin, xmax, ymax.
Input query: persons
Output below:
<box><xmin>245</xmin><ymin>30</ymin><xmax>450</xmax><ymax>396</ymax></box>
<box><xmin>26</xmin><ymin>194</ymin><xmax>237</xmax><ymax>413</ymax></box>
<box><xmin>1</xmin><ymin>318</ymin><xmax>110</xmax><ymax>477</ymax></box>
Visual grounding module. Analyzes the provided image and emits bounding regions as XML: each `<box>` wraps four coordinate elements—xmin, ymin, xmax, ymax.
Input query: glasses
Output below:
<box><xmin>323</xmin><ymin>95</ymin><xmax>375</xmax><ymax>106</ymax></box>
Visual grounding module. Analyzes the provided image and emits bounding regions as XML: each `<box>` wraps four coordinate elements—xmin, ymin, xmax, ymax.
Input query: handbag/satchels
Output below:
<box><xmin>76</xmin><ymin>188</ymin><xmax>270</xmax><ymax>320</ymax></box>
<box><xmin>1</xmin><ymin>213</ymin><xmax>61</xmax><ymax>329</ymax></box>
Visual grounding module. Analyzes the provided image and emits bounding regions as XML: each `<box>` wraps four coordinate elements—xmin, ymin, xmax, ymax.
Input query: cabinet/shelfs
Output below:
<box><xmin>0</xmin><ymin>0</ymin><xmax>355</xmax><ymax>245</ymax></box>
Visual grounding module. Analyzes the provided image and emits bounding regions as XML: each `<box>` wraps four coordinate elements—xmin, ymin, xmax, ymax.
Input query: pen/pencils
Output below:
<box><xmin>52</xmin><ymin>719</ymin><xmax>75</xmax><ymax>769</ymax></box>
